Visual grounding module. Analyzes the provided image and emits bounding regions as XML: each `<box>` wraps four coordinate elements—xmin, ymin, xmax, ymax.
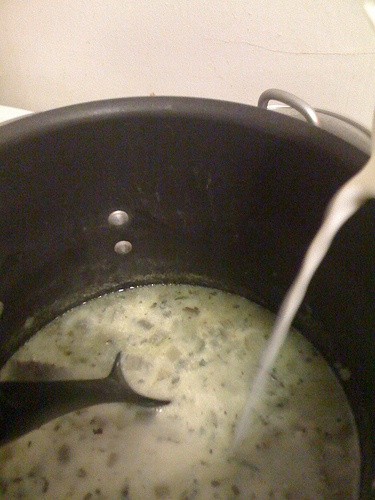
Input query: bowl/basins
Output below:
<box><xmin>0</xmin><ymin>95</ymin><xmax>374</xmax><ymax>499</ymax></box>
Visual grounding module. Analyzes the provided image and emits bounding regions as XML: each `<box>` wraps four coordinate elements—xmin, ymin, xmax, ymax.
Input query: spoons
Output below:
<box><xmin>0</xmin><ymin>348</ymin><xmax>173</xmax><ymax>447</ymax></box>
